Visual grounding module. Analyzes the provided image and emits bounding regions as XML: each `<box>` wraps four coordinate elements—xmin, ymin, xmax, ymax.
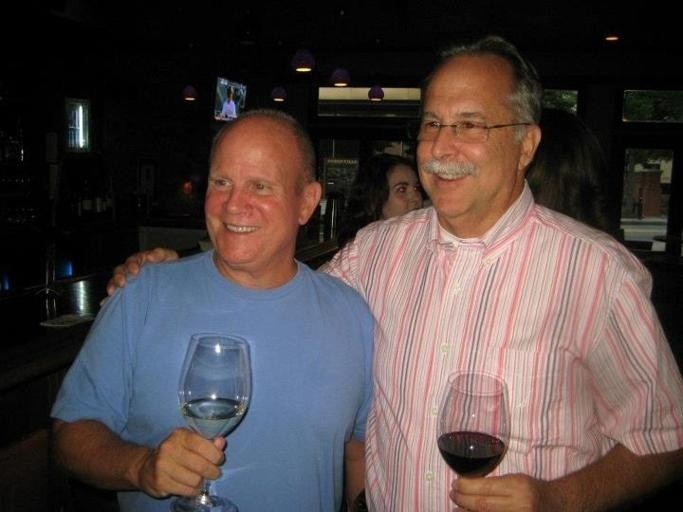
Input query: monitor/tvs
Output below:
<box><xmin>208</xmin><ymin>72</ymin><xmax>253</xmax><ymax>124</ymax></box>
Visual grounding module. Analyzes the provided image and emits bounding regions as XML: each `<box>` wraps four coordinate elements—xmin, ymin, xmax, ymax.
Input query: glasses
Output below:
<box><xmin>408</xmin><ymin>119</ymin><xmax>529</xmax><ymax>143</ymax></box>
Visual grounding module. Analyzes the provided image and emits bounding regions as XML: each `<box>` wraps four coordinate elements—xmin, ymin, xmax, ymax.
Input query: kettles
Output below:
<box><xmin>324</xmin><ymin>191</ymin><xmax>344</xmax><ymax>229</ymax></box>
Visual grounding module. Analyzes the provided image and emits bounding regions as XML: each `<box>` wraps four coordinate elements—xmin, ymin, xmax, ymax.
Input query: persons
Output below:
<box><xmin>219</xmin><ymin>85</ymin><xmax>238</xmax><ymax>118</ymax></box>
<box><xmin>335</xmin><ymin>154</ymin><xmax>421</xmax><ymax>242</ymax></box>
<box><xmin>49</xmin><ymin>108</ymin><xmax>376</xmax><ymax>511</ymax></box>
<box><xmin>100</xmin><ymin>32</ymin><xmax>682</xmax><ymax>510</ymax></box>
<box><xmin>524</xmin><ymin>104</ymin><xmax>632</xmax><ymax>254</ymax></box>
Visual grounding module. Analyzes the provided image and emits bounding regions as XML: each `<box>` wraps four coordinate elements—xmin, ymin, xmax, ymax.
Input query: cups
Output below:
<box><xmin>318</xmin><ymin>201</ymin><xmax>326</xmax><ymax>216</ymax></box>
<box><xmin>434</xmin><ymin>370</ymin><xmax>509</xmax><ymax>478</ymax></box>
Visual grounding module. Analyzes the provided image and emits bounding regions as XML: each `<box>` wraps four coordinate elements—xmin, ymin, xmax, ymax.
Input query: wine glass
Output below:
<box><xmin>167</xmin><ymin>333</ymin><xmax>251</xmax><ymax>511</ymax></box>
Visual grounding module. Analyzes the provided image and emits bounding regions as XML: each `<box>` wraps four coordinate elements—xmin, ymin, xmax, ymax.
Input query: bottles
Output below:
<box><xmin>0</xmin><ymin>207</ymin><xmax>36</xmax><ymax>225</ymax></box>
<box><xmin>69</xmin><ymin>175</ymin><xmax>114</xmax><ymax>222</ymax></box>
<box><xmin>143</xmin><ymin>167</ymin><xmax>152</xmax><ymax>197</ymax></box>
